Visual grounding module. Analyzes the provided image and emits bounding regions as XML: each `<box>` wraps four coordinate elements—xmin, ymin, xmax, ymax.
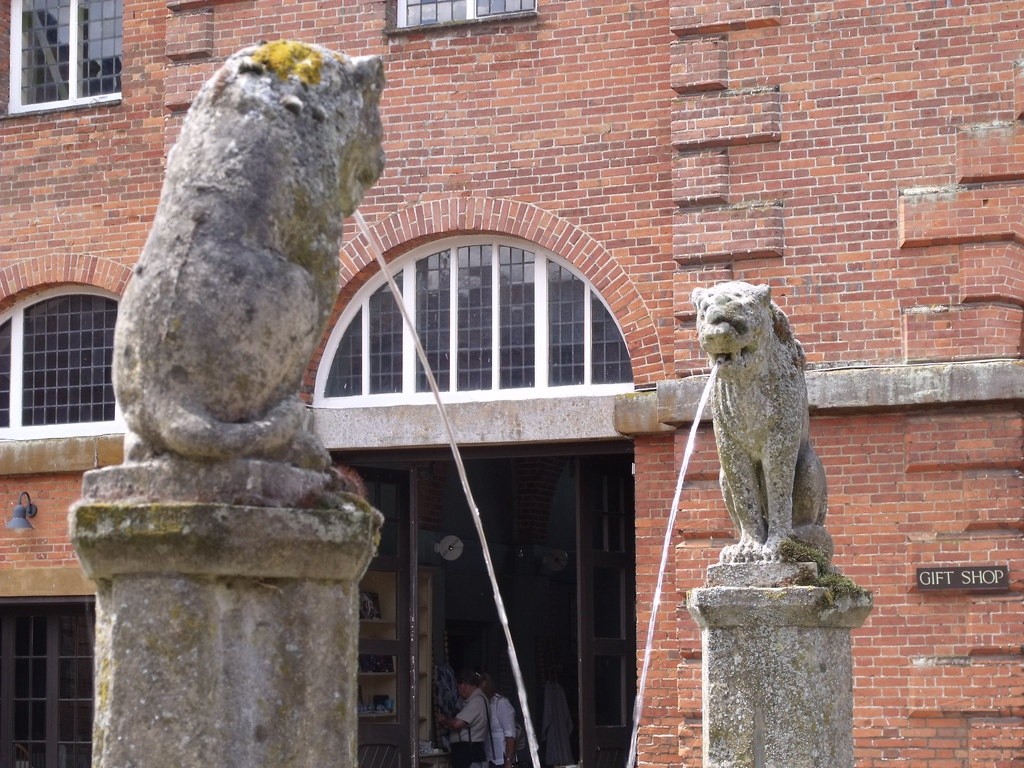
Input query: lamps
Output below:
<box><xmin>6</xmin><ymin>490</ymin><xmax>37</xmax><ymax>535</ymax></box>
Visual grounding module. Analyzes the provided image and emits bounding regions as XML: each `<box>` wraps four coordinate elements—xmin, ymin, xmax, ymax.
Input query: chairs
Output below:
<box><xmin>358</xmin><ymin>742</ymin><xmax>401</xmax><ymax>768</ymax></box>
<box><xmin>13</xmin><ymin>743</ymin><xmax>89</xmax><ymax>768</ymax></box>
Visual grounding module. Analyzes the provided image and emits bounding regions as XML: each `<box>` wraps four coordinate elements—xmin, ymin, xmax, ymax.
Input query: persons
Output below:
<box><xmin>435</xmin><ymin>667</ymin><xmax>491</xmax><ymax>768</ymax></box>
<box><xmin>472</xmin><ymin>671</ymin><xmax>519</xmax><ymax>768</ymax></box>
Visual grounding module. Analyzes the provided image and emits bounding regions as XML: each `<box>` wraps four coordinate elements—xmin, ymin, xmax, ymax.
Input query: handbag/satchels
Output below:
<box><xmin>450</xmin><ymin>726</ymin><xmax>486</xmax><ymax>763</ymax></box>
<box><xmin>489</xmin><ymin>758</ymin><xmax>505</xmax><ymax>767</ymax></box>
